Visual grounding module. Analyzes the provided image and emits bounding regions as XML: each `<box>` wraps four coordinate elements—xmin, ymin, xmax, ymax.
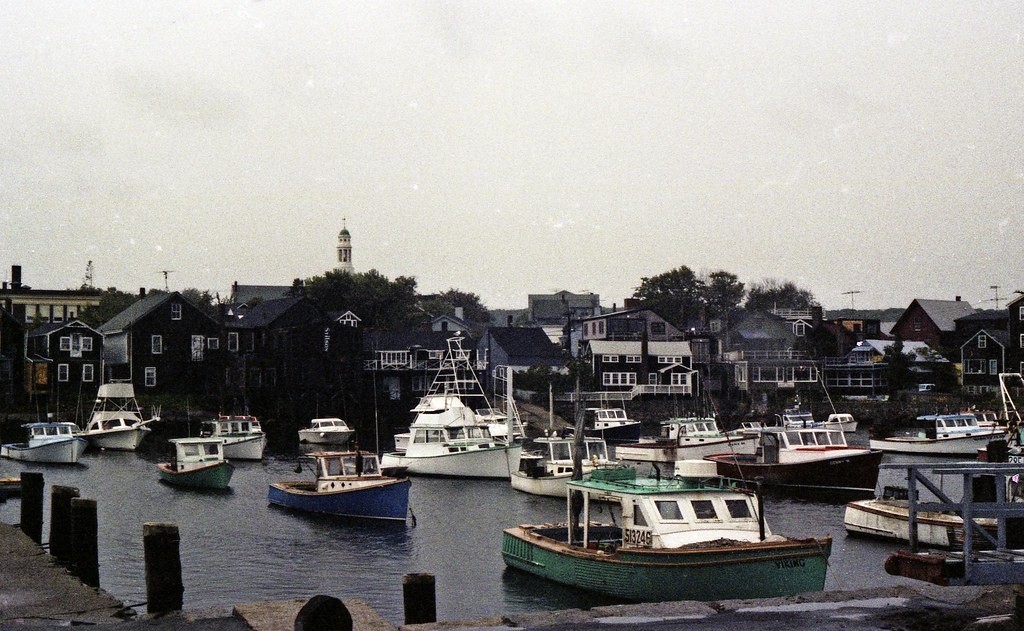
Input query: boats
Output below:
<box><xmin>510</xmin><ymin>430</ymin><xmax>609</xmax><ymax>498</ymax></box>
<box><xmin>381</xmin><ymin>336</ymin><xmax>529</xmax><ymax>480</ymax></box>
<box><xmin>674</xmin><ymin>431</ymin><xmax>885</xmax><ymax>490</ymax></box>
<box><xmin>198</xmin><ymin>414</ymin><xmax>269</xmax><ymax>462</ymax></box>
<box><xmin>843</xmin><ymin>420</ymin><xmax>1024</xmax><ymax>551</ymax></box>
<box><xmin>0</xmin><ymin>413</ymin><xmax>91</xmax><ymax>464</ymax></box>
<box><xmin>585</xmin><ymin>393</ymin><xmax>858</xmax><ymax>463</ymax></box>
<box><xmin>267</xmin><ymin>445</ymin><xmax>412</xmax><ymax>522</ymax></box>
<box><xmin>874</xmin><ymin>460</ymin><xmax>1024</xmax><ymax>604</ymax></box>
<box><xmin>507</xmin><ymin>451</ymin><xmax>833</xmax><ymax>605</ymax></box>
<box><xmin>85</xmin><ymin>383</ymin><xmax>164</xmax><ymax>450</ymax></box>
<box><xmin>157</xmin><ymin>437</ymin><xmax>237</xmax><ymax>491</ymax></box>
<box><xmin>868</xmin><ymin>410</ymin><xmax>1017</xmax><ymax>456</ymax></box>
<box><xmin>298</xmin><ymin>418</ymin><xmax>356</xmax><ymax>447</ymax></box>
